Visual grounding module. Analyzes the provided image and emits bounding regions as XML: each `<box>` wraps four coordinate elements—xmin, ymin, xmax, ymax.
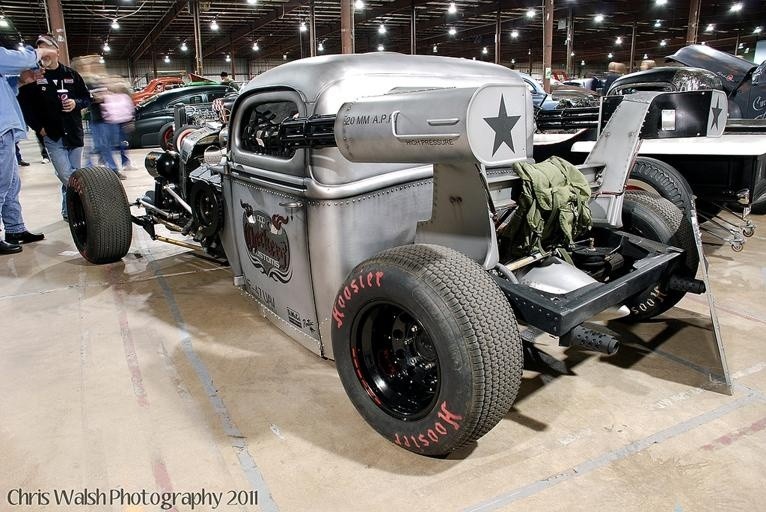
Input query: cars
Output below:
<box><xmin>58</xmin><ymin>50</ymin><xmax>752</xmax><ymax>458</ymax></box>
<box><xmin>515</xmin><ymin>66</ymin><xmax>603</xmax><ymax>135</ymax></box>
<box><xmin>121</xmin><ymin>85</ymin><xmax>238</xmax><ymax>150</ymax></box>
<box><xmin>604</xmin><ymin>43</ymin><xmax>766</xmax><ymax>133</ymax></box>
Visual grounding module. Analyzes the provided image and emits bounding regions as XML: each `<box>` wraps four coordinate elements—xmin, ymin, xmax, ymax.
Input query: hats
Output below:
<box><xmin>36</xmin><ymin>35</ymin><xmax>59</xmax><ymax>48</ymax></box>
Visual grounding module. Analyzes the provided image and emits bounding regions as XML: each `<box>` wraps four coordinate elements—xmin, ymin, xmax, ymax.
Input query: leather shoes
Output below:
<box><xmin>0</xmin><ymin>241</ymin><xmax>22</xmax><ymax>254</ymax></box>
<box><xmin>18</xmin><ymin>159</ymin><xmax>30</xmax><ymax>165</ymax></box>
<box><xmin>5</xmin><ymin>231</ymin><xmax>44</xmax><ymax>244</ymax></box>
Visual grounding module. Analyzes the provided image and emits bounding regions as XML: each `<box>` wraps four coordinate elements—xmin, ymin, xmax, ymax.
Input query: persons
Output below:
<box><xmin>0</xmin><ymin>19</ymin><xmax>134</xmax><ymax>254</ymax></box>
<box><xmin>605</xmin><ymin>59</ymin><xmax>656</xmax><ymax>94</ymax></box>
<box><xmin>219</xmin><ymin>71</ymin><xmax>239</xmax><ymax>89</ymax></box>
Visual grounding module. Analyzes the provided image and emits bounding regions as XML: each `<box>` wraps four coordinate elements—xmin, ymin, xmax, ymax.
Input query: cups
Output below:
<box><xmin>57</xmin><ymin>89</ymin><xmax>69</xmax><ymax>112</ymax></box>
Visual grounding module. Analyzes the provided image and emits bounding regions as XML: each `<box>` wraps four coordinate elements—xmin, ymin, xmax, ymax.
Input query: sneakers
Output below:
<box><xmin>41</xmin><ymin>158</ymin><xmax>49</xmax><ymax>163</ymax></box>
<box><xmin>113</xmin><ymin>165</ymin><xmax>137</xmax><ymax>180</ymax></box>
